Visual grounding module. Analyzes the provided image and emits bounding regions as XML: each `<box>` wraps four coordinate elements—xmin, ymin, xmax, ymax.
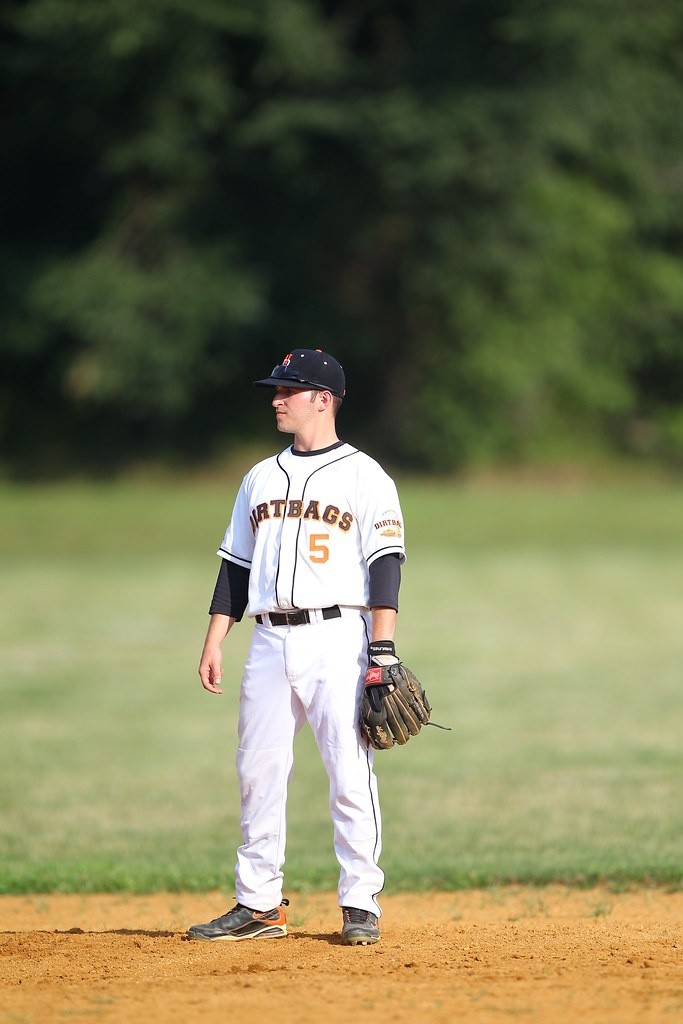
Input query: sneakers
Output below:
<box><xmin>188</xmin><ymin>902</ymin><xmax>289</xmax><ymax>942</ymax></box>
<box><xmin>340</xmin><ymin>906</ymin><xmax>381</xmax><ymax>945</ymax></box>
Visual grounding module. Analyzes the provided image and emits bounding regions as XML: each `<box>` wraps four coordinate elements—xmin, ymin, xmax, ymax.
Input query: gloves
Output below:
<box><xmin>367</xmin><ymin>640</ymin><xmax>399</xmax><ymax>713</ymax></box>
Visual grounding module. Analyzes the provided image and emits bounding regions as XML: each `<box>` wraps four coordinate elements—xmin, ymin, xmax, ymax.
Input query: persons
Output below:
<box><xmin>182</xmin><ymin>348</ymin><xmax>453</xmax><ymax>947</ymax></box>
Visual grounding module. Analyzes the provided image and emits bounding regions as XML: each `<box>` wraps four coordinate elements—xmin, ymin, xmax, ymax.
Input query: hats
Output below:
<box><xmin>253</xmin><ymin>349</ymin><xmax>346</xmax><ymax>400</ymax></box>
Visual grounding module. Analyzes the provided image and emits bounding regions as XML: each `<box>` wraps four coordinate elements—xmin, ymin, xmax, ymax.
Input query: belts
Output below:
<box><xmin>255</xmin><ymin>604</ymin><xmax>342</xmax><ymax>626</ymax></box>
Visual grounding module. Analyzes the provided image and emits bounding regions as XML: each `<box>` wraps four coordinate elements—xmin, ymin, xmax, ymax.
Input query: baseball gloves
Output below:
<box><xmin>360</xmin><ymin>641</ymin><xmax>453</xmax><ymax>750</ymax></box>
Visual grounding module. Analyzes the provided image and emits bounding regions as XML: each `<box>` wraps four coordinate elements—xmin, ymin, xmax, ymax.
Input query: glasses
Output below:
<box><xmin>270</xmin><ymin>362</ymin><xmax>335</xmax><ymax>393</ymax></box>
